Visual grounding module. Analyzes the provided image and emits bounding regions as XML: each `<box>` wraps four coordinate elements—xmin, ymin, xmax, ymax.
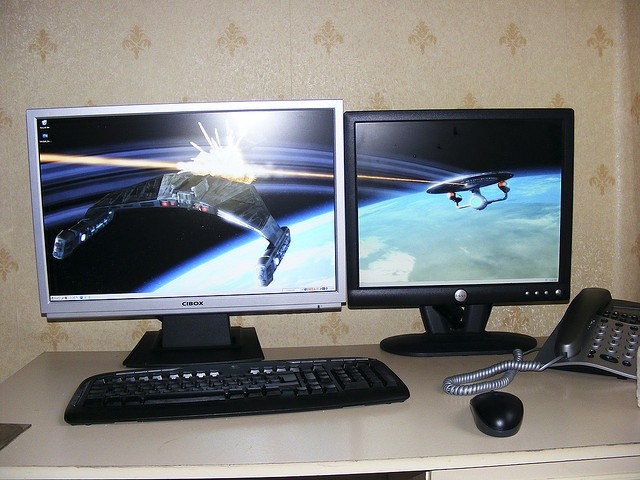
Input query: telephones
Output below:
<box><xmin>442</xmin><ymin>287</ymin><xmax>640</xmax><ymax>396</ymax></box>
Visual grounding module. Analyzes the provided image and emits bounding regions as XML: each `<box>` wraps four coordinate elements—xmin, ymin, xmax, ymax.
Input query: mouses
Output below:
<box><xmin>470</xmin><ymin>390</ymin><xmax>524</xmax><ymax>438</ymax></box>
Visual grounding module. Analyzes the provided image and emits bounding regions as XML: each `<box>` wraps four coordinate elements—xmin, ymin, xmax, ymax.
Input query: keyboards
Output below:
<box><xmin>62</xmin><ymin>357</ymin><xmax>410</xmax><ymax>424</ymax></box>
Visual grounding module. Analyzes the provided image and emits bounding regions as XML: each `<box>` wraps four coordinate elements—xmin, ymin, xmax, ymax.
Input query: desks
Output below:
<box><xmin>2</xmin><ymin>341</ymin><xmax>639</xmax><ymax>480</ymax></box>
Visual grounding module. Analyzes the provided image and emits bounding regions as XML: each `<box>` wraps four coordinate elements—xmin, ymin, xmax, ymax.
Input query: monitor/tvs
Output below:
<box><xmin>25</xmin><ymin>99</ymin><xmax>348</xmax><ymax>368</ymax></box>
<box><xmin>343</xmin><ymin>108</ymin><xmax>574</xmax><ymax>357</ymax></box>
